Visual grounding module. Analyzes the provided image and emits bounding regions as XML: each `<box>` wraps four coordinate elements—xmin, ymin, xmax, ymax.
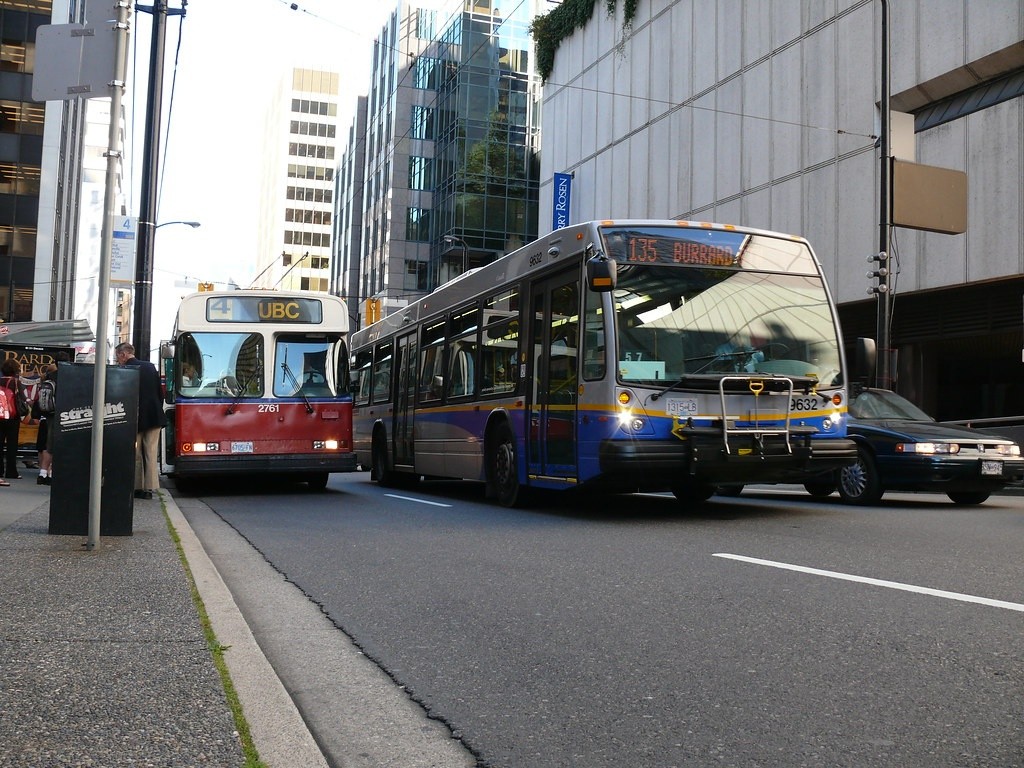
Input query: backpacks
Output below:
<box><xmin>0</xmin><ymin>377</ymin><xmax>17</xmax><ymax>420</ymax></box>
<box><xmin>37</xmin><ymin>380</ymin><xmax>56</xmax><ymax>414</ymax></box>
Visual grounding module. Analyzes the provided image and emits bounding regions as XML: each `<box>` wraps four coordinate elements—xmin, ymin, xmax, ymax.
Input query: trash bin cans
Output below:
<box><xmin>49</xmin><ymin>362</ymin><xmax>143</xmax><ymax>535</ymax></box>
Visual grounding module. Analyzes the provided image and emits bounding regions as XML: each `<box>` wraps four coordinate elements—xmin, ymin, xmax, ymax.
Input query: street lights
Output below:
<box><xmin>442</xmin><ymin>234</ymin><xmax>468</xmax><ymax>273</ymax></box>
<box><xmin>139</xmin><ymin>221</ymin><xmax>202</xmax><ymax>362</ymax></box>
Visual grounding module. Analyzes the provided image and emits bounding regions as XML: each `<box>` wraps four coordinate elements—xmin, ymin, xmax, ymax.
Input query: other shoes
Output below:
<box><xmin>17</xmin><ymin>475</ymin><xmax>22</xmax><ymax>478</ymax></box>
<box><xmin>0</xmin><ymin>477</ymin><xmax>10</xmax><ymax>485</ymax></box>
<box><xmin>143</xmin><ymin>489</ymin><xmax>152</xmax><ymax>499</ymax></box>
<box><xmin>134</xmin><ymin>489</ymin><xmax>145</xmax><ymax>498</ymax></box>
<box><xmin>37</xmin><ymin>475</ymin><xmax>52</xmax><ymax>486</ymax></box>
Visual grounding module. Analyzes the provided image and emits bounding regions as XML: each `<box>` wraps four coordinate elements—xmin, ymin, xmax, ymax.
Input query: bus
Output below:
<box><xmin>347</xmin><ymin>218</ymin><xmax>859</xmax><ymax>515</ymax></box>
<box><xmin>159</xmin><ymin>251</ymin><xmax>357</xmax><ymax>494</ymax></box>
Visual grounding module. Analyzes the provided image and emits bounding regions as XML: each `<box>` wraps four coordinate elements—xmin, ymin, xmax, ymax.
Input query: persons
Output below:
<box><xmin>281</xmin><ymin>354</ymin><xmax>325</xmax><ymax>383</ymax></box>
<box><xmin>167</xmin><ymin>363</ymin><xmax>195</xmax><ymax>454</ymax></box>
<box><xmin>0</xmin><ymin>352</ymin><xmax>69</xmax><ymax>486</ymax></box>
<box><xmin>714</xmin><ymin>328</ymin><xmax>764</xmax><ymax>373</ymax></box>
<box><xmin>115</xmin><ymin>342</ymin><xmax>167</xmax><ymax>499</ymax></box>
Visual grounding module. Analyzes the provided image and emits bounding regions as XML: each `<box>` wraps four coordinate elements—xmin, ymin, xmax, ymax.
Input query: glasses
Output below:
<box><xmin>191</xmin><ymin>371</ymin><xmax>195</xmax><ymax>375</ymax></box>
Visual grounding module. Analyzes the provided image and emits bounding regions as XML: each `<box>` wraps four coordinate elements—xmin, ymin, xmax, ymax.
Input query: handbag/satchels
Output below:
<box><xmin>15</xmin><ymin>378</ymin><xmax>29</xmax><ymax>416</ymax></box>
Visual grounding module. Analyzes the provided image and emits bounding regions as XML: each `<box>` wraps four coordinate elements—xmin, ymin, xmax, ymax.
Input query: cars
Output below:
<box><xmin>715</xmin><ymin>385</ymin><xmax>1024</xmax><ymax>509</ymax></box>
